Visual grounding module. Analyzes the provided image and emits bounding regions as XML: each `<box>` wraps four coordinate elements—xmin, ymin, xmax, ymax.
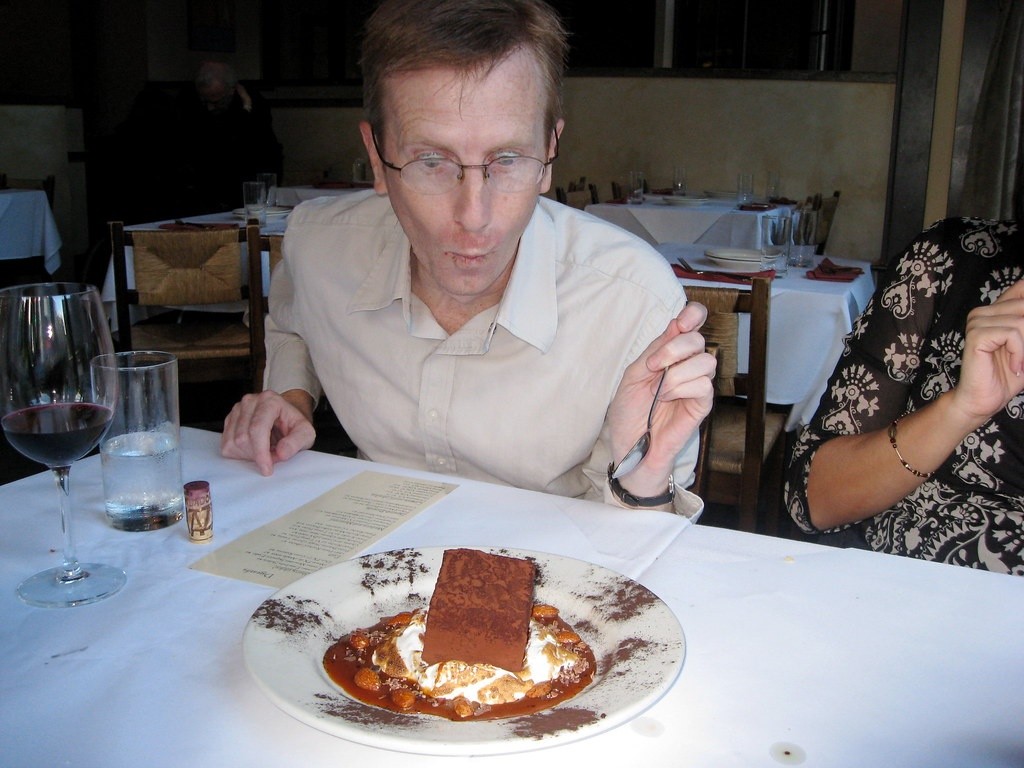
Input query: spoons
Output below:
<box><xmin>612</xmin><ymin>300</ymin><xmax>689</xmax><ymax>478</ymax></box>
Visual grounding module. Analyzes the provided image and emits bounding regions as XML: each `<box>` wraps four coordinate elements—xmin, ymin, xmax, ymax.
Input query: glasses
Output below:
<box><xmin>371</xmin><ymin>121</ymin><xmax>559</xmax><ymax>196</ymax></box>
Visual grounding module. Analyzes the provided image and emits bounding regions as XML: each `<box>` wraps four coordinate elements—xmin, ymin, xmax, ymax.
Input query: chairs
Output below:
<box><xmin>0</xmin><ymin>173</ymin><xmax>841</xmax><ymax>543</ymax></box>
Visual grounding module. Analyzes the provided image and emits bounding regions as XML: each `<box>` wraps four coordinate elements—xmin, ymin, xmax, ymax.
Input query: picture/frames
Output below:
<box><xmin>187</xmin><ymin>0</ymin><xmax>236</xmax><ymax>52</ymax></box>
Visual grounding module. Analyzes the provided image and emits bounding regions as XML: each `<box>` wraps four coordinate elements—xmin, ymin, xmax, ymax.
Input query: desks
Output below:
<box><xmin>0</xmin><ymin>420</ymin><xmax>1024</xmax><ymax>768</ymax></box>
<box><xmin>266</xmin><ymin>184</ymin><xmax>376</xmax><ymax>207</ymax></box>
<box><xmin>98</xmin><ymin>206</ymin><xmax>287</xmax><ymax>338</ymax></box>
<box><xmin>0</xmin><ymin>186</ymin><xmax>63</xmax><ymax>289</ymax></box>
<box><xmin>655</xmin><ymin>243</ymin><xmax>875</xmax><ymax>432</ymax></box>
<box><xmin>582</xmin><ymin>193</ymin><xmax>800</xmax><ymax>250</ymax></box>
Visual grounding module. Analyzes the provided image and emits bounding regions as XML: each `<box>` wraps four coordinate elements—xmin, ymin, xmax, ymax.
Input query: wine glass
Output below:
<box><xmin>0</xmin><ymin>282</ymin><xmax>119</xmax><ymax>609</ymax></box>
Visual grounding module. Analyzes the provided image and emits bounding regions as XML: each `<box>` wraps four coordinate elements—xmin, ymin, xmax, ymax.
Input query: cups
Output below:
<box><xmin>759</xmin><ymin>215</ymin><xmax>792</xmax><ymax>279</ymax></box>
<box><xmin>765</xmin><ymin>167</ymin><xmax>780</xmax><ymax>202</ymax></box>
<box><xmin>737</xmin><ymin>174</ymin><xmax>753</xmax><ymax>207</ymax></box>
<box><xmin>628</xmin><ymin>171</ymin><xmax>644</xmax><ymax>204</ymax></box>
<box><xmin>672</xmin><ymin>165</ymin><xmax>687</xmax><ymax>197</ymax></box>
<box><xmin>352</xmin><ymin>162</ymin><xmax>366</xmax><ymax>182</ymax></box>
<box><xmin>789</xmin><ymin>209</ymin><xmax>817</xmax><ymax>268</ymax></box>
<box><xmin>242</xmin><ymin>173</ymin><xmax>277</xmax><ymax>229</ymax></box>
<box><xmin>89</xmin><ymin>351</ymin><xmax>185</xmax><ymax>532</ymax></box>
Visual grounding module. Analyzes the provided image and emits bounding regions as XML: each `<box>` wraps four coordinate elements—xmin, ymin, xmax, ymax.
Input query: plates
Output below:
<box><xmin>230</xmin><ymin>207</ymin><xmax>292</xmax><ymax>220</ymax></box>
<box><xmin>704</xmin><ymin>246</ymin><xmax>780</xmax><ymax>271</ymax></box>
<box><xmin>240</xmin><ymin>545</ymin><xmax>687</xmax><ymax>757</ymax></box>
<box><xmin>662</xmin><ymin>196</ymin><xmax>712</xmax><ymax>205</ymax></box>
<box><xmin>701</xmin><ymin>190</ymin><xmax>738</xmax><ymax>199</ymax></box>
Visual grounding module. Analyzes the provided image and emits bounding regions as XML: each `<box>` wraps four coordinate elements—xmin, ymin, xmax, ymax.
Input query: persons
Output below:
<box><xmin>758</xmin><ymin>210</ymin><xmax>1024</xmax><ymax>577</ymax></box>
<box><xmin>216</xmin><ymin>0</ymin><xmax>721</xmax><ymax>523</ymax></box>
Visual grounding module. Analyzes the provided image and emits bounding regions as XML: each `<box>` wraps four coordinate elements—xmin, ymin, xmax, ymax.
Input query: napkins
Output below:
<box><xmin>652</xmin><ymin>187</ymin><xmax>672</xmax><ymax>195</ymax></box>
<box><xmin>770</xmin><ymin>198</ymin><xmax>797</xmax><ymax>204</ymax></box>
<box><xmin>159</xmin><ymin>221</ymin><xmax>232</xmax><ymax>230</ymax></box>
<box><xmin>312</xmin><ymin>178</ymin><xmax>367</xmax><ymax>188</ymax></box>
<box><xmin>606</xmin><ymin>192</ymin><xmax>646</xmax><ymax>204</ymax></box>
<box><xmin>741</xmin><ymin>202</ymin><xmax>776</xmax><ymax>212</ymax></box>
<box><xmin>806</xmin><ymin>258</ymin><xmax>863</xmax><ymax>281</ymax></box>
<box><xmin>672</xmin><ymin>264</ymin><xmax>774</xmax><ymax>285</ymax></box>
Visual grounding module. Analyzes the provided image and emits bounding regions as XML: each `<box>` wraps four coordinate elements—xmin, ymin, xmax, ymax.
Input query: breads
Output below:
<box><xmin>421</xmin><ymin>548</ymin><xmax>538</xmax><ymax>672</ymax></box>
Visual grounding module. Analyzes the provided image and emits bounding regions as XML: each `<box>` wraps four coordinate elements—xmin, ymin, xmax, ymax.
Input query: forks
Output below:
<box><xmin>676</xmin><ymin>256</ymin><xmax>752</xmax><ymax>282</ymax></box>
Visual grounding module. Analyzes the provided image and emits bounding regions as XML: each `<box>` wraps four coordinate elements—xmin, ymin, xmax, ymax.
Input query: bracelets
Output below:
<box><xmin>606</xmin><ymin>461</ymin><xmax>675</xmax><ymax>507</ymax></box>
<box><xmin>887</xmin><ymin>410</ymin><xmax>934</xmax><ymax>478</ymax></box>
